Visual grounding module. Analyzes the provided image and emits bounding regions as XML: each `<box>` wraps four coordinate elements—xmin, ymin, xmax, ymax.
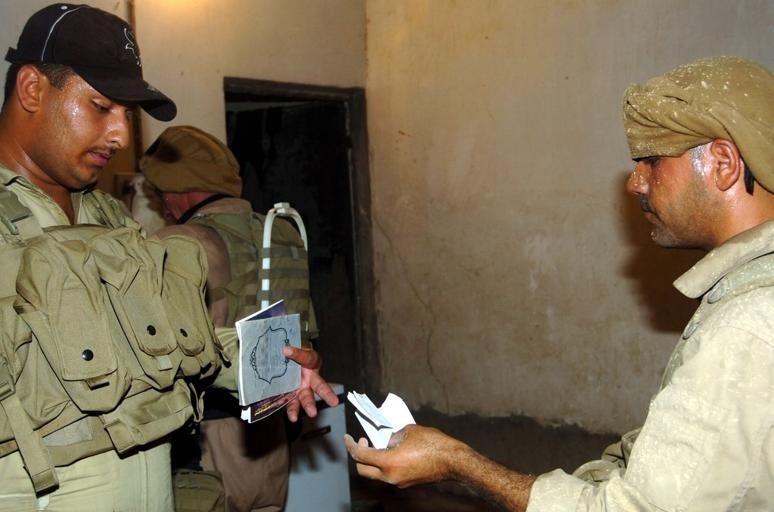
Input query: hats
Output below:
<box><xmin>138</xmin><ymin>126</ymin><xmax>242</xmax><ymax>197</ymax></box>
<box><xmin>6</xmin><ymin>3</ymin><xmax>177</xmax><ymax>122</ymax></box>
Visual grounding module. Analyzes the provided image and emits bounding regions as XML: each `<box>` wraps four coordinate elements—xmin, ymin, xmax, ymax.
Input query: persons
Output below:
<box><xmin>343</xmin><ymin>56</ymin><xmax>774</xmax><ymax>512</ymax></box>
